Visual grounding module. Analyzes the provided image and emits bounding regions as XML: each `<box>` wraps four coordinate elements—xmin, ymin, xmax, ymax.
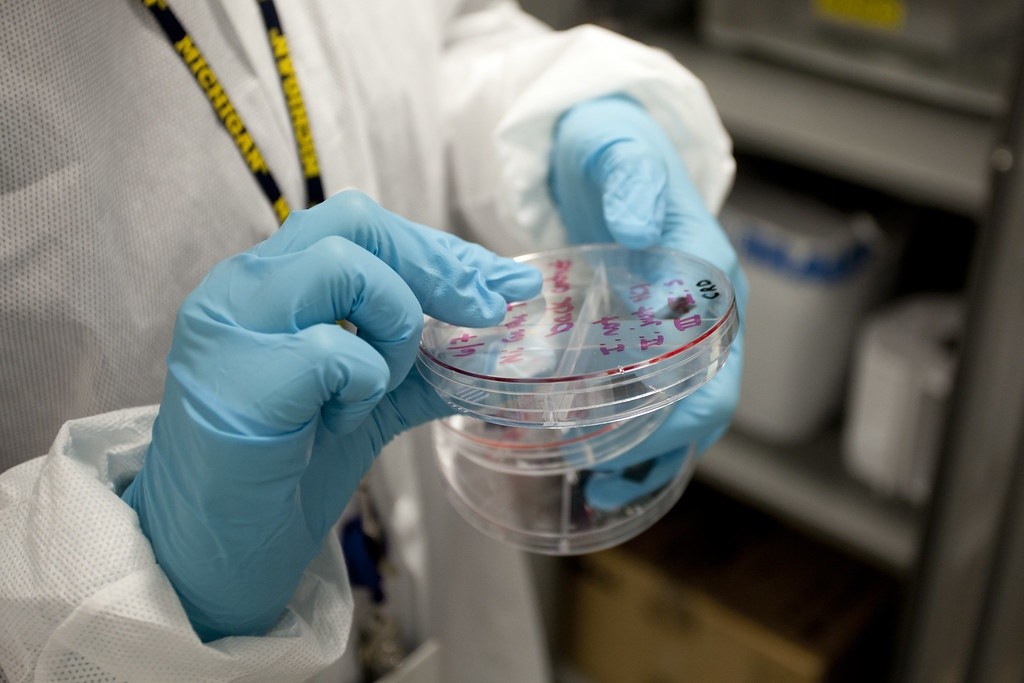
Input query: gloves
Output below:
<box><xmin>119</xmin><ymin>190</ymin><xmax>544</xmax><ymax>645</ymax></box>
<box><xmin>540</xmin><ymin>91</ymin><xmax>748</xmax><ymax>524</ymax></box>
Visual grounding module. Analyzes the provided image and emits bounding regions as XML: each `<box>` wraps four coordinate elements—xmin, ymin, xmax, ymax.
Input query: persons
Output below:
<box><xmin>0</xmin><ymin>0</ymin><xmax>737</xmax><ymax>683</ymax></box>
